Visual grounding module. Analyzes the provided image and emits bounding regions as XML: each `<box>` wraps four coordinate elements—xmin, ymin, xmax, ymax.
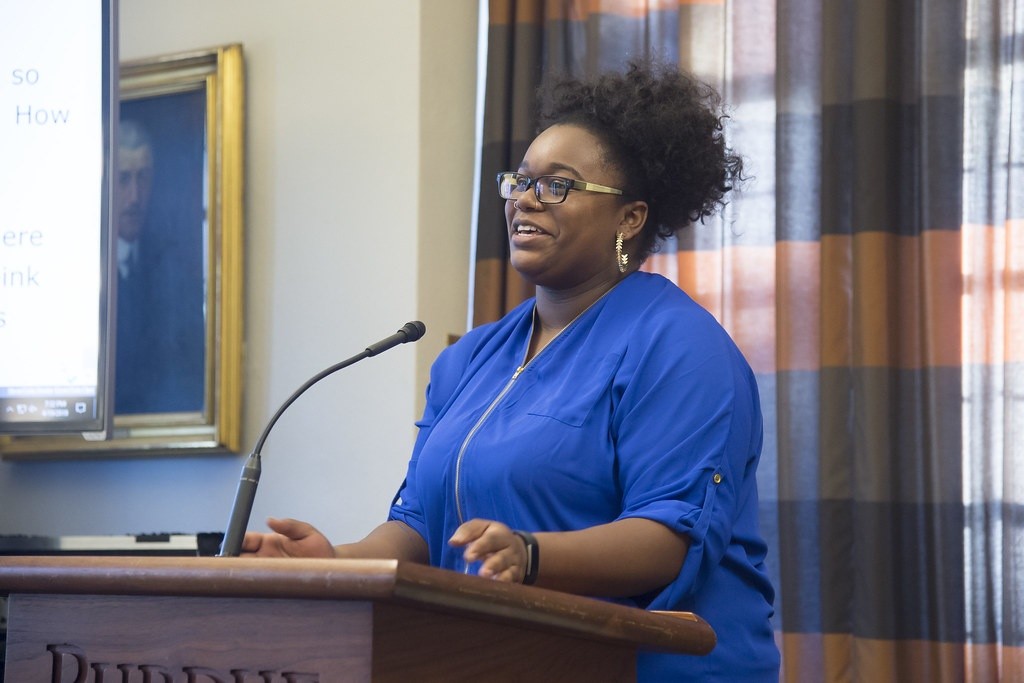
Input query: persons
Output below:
<box><xmin>238</xmin><ymin>63</ymin><xmax>782</xmax><ymax>683</ymax></box>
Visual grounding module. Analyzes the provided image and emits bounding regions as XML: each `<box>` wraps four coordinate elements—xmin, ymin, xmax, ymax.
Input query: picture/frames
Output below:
<box><xmin>0</xmin><ymin>43</ymin><xmax>244</xmax><ymax>457</ymax></box>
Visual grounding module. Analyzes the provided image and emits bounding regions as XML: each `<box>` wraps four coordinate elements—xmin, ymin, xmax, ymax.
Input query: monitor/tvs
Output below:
<box><xmin>0</xmin><ymin>0</ymin><xmax>120</xmax><ymax>441</ymax></box>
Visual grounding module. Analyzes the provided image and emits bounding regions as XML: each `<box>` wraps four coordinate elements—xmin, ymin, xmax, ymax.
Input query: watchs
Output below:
<box><xmin>513</xmin><ymin>529</ymin><xmax>539</xmax><ymax>586</ymax></box>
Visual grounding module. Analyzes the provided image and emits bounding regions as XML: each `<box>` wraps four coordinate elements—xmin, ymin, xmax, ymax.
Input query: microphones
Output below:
<box><xmin>215</xmin><ymin>321</ymin><xmax>426</xmax><ymax>558</ymax></box>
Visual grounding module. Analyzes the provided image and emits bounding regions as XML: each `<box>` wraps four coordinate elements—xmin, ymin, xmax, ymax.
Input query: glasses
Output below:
<box><xmin>497</xmin><ymin>171</ymin><xmax>625</xmax><ymax>204</ymax></box>
